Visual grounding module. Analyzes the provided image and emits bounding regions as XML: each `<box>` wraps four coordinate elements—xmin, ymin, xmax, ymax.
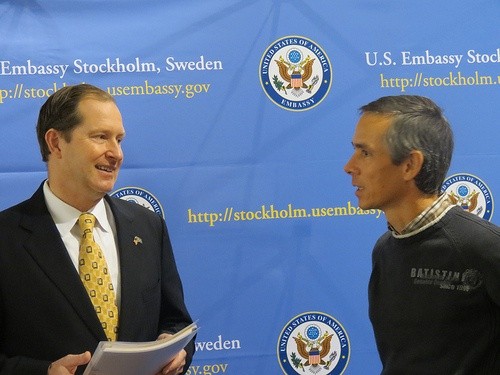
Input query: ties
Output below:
<box><xmin>77</xmin><ymin>213</ymin><xmax>118</xmax><ymax>342</ymax></box>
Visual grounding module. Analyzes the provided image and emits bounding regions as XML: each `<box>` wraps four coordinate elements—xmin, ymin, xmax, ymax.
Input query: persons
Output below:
<box><xmin>345</xmin><ymin>95</ymin><xmax>500</xmax><ymax>375</ymax></box>
<box><xmin>1</xmin><ymin>84</ymin><xmax>197</xmax><ymax>375</ymax></box>
